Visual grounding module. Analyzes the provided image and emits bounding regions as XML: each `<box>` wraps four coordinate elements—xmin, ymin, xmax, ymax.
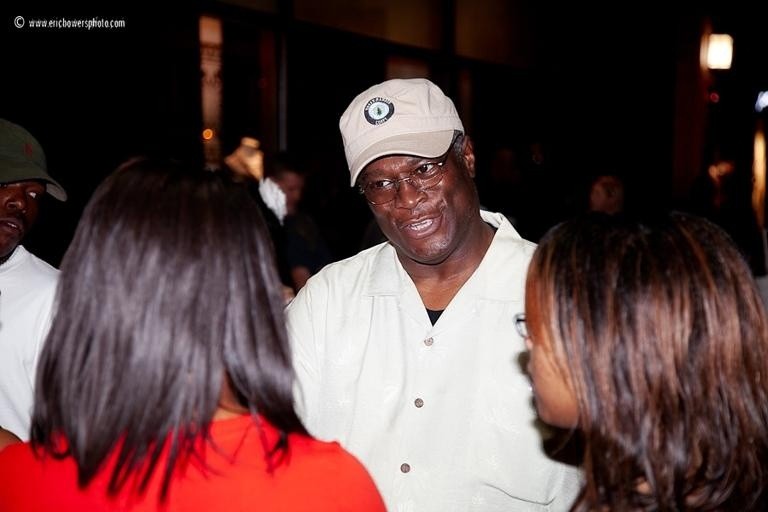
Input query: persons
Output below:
<box><xmin>0</xmin><ymin>153</ymin><xmax>386</xmax><ymax>511</ymax></box>
<box><xmin>514</xmin><ymin>206</ymin><xmax>768</xmax><ymax>511</ymax></box>
<box><xmin>280</xmin><ymin>75</ymin><xmax>587</xmax><ymax>511</ymax></box>
<box><xmin>252</xmin><ymin>155</ymin><xmax>312</xmax><ymax>307</ymax></box>
<box><xmin>0</xmin><ymin>116</ymin><xmax>62</xmax><ymax>445</ymax></box>
<box><xmin>528</xmin><ymin>140</ymin><xmax>547</xmax><ymax>166</ymax></box>
<box><xmin>576</xmin><ymin>174</ymin><xmax>636</xmax><ymax>231</ymax></box>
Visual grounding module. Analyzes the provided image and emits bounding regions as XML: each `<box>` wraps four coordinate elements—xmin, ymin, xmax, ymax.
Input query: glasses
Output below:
<box><xmin>358</xmin><ymin>132</ymin><xmax>461</xmax><ymax>205</ymax></box>
<box><xmin>513</xmin><ymin>313</ymin><xmax>528</xmax><ymax>338</ymax></box>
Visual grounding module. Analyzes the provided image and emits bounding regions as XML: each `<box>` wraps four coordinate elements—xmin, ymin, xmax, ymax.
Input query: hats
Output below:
<box><xmin>339</xmin><ymin>79</ymin><xmax>465</xmax><ymax>187</ymax></box>
<box><xmin>0</xmin><ymin>120</ymin><xmax>68</xmax><ymax>201</ymax></box>
